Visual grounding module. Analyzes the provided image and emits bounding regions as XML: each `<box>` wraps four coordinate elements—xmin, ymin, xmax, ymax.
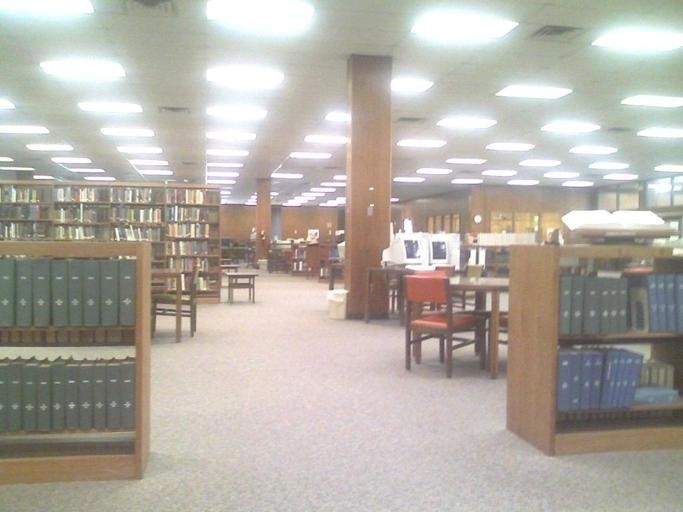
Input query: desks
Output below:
<box><xmin>150</xmin><ymin>267</ymin><xmax>194</xmax><ymax>343</ymax></box>
<box><xmin>366</xmin><ymin>266</ymin><xmax>405</xmax><ymax>320</ymax></box>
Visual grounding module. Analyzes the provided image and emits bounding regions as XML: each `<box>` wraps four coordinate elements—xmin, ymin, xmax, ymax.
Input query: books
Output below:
<box><xmin>109</xmin><ymin>188</ymin><xmax>160</xmax><ymax>268</ymax></box>
<box><xmin>554</xmin><ymin>268</ymin><xmax>682</xmax><ymax>412</ymax></box>
<box><xmin>52</xmin><ymin>186</ymin><xmax>103</xmax><ymax>241</ymax></box>
<box><xmin>165</xmin><ymin>189</ymin><xmax>210</xmax><ymax>292</ymax></box>
<box><xmin>1</xmin><ymin>185</ymin><xmax>45</xmax><ymax>240</ymax></box>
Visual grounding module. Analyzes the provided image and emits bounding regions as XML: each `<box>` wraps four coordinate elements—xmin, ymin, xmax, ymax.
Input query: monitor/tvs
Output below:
<box><xmin>381</xmin><ymin>233</ymin><xmax>424</xmax><ymax>263</ymax></box>
<box><xmin>427</xmin><ymin>235</ymin><xmax>451</xmax><ymax>267</ymax></box>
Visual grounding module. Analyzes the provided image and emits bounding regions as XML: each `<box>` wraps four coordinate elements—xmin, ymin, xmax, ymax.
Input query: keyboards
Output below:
<box><xmin>406</xmin><ymin>265</ymin><xmax>438</xmax><ymax>271</ymax></box>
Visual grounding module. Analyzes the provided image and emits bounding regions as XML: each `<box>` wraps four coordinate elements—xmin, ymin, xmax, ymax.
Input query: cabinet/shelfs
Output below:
<box><xmin>0</xmin><ymin>179</ymin><xmax>223</xmax><ymax>305</ymax></box>
<box><xmin>266</xmin><ymin>237</ymin><xmax>347</xmax><ymax>290</ymax></box>
<box><xmin>0</xmin><ymin>240</ymin><xmax>149</xmax><ymax>482</ymax></box>
<box><xmin>507</xmin><ymin>244</ymin><xmax>683</xmax><ymax>456</ymax></box>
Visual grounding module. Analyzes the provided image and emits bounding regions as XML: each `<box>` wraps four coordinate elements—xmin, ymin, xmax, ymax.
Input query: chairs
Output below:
<box><xmin>150</xmin><ymin>255</ymin><xmax>201</xmax><ymax>343</ymax></box>
<box><xmin>403</xmin><ymin>264</ymin><xmax>509</xmax><ymax>378</ymax></box>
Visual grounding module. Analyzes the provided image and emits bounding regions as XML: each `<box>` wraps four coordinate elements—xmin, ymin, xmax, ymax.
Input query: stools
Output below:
<box><xmin>220</xmin><ymin>257</ymin><xmax>260</xmax><ymax>304</ymax></box>
